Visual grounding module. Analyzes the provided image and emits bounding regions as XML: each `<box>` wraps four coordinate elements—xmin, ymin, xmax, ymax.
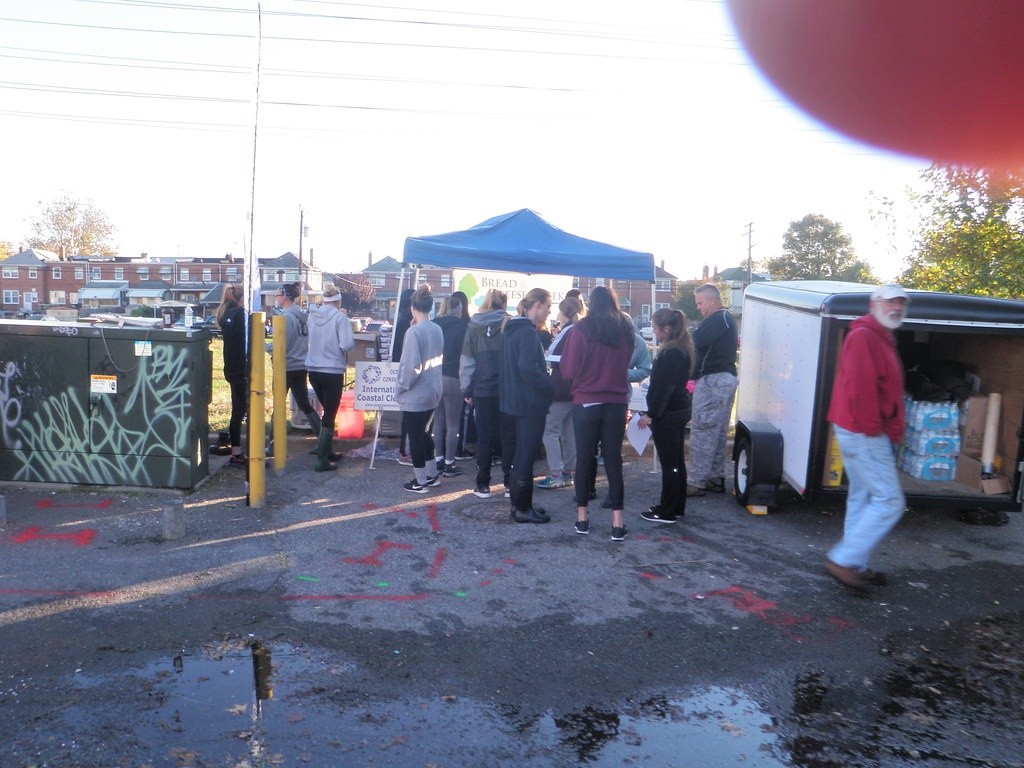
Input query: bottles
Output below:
<box><xmin>893</xmin><ymin>398</ymin><xmax>963</xmax><ymax>482</ymax></box>
<box><xmin>161</xmin><ymin>308</ymin><xmax>174</xmax><ymax>327</ymax></box>
<box><xmin>184</xmin><ymin>304</ymin><xmax>194</xmax><ymax>327</ymax></box>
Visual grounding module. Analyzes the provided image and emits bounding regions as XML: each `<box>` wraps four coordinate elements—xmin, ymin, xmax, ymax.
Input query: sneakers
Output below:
<box><xmin>641</xmin><ymin>505</ymin><xmax>681</xmax><ymax>523</ymax></box>
<box><xmin>537</xmin><ymin>470</ymin><xmax>565</xmax><ymax>488</ymax></box>
<box><xmin>563</xmin><ymin>471</ymin><xmax>573</xmax><ymax>485</ymax></box>
<box><xmin>398</xmin><ymin>449</ymin><xmax>511</xmax><ymax>498</ymax></box>
<box><xmin>611</xmin><ymin>523</ymin><xmax>630</xmax><ymax>540</ymax></box>
<box><xmin>574</xmin><ymin>517</ymin><xmax>590</xmax><ymax>534</ymax></box>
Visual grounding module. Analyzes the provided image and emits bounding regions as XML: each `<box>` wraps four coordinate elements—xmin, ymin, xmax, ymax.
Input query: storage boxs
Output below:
<box><xmin>826</xmin><ymin>431</ymin><xmax>844</xmax><ymax>486</ymax></box>
<box><xmin>958</xmin><ymin>392</ymin><xmax>989</xmax><ymax>456</ymax></box>
<box><xmin>953</xmin><ymin>453</ymin><xmax>1013</xmax><ymax>495</ymax></box>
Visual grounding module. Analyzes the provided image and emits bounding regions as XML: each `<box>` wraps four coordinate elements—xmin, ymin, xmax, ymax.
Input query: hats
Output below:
<box><xmin>872</xmin><ymin>283</ymin><xmax>911</xmax><ymax>302</ymax></box>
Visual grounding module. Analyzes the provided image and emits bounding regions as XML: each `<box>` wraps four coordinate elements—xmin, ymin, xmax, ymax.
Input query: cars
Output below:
<box><xmin>191</xmin><ymin>315</ymin><xmax>223</xmax><ymax>338</ymax></box>
<box><xmin>639</xmin><ymin>320</ymin><xmax>653</xmax><ymax>341</ymax></box>
<box><xmin>172</xmin><ymin>316</ymin><xmax>204</xmax><ymax>328</ymax></box>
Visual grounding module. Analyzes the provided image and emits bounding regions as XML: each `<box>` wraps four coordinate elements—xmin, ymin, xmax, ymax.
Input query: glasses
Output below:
<box><xmin>274</xmin><ymin>294</ymin><xmax>290</xmax><ymax>300</ymax></box>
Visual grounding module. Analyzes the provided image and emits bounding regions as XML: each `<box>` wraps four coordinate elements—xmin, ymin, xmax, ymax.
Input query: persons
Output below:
<box><xmin>497</xmin><ymin>288</ymin><xmax>554</xmax><ymax>524</ymax></box>
<box><xmin>264</xmin><ymin>282</ymin><xmax>321</xmax><ymax>457</ymax></box>
<box><xmin>686</xmin><ymin>285</ymin><xmax>737</xmax><ymax>497</ymax></box>
<box><xmin>638</xmin><ymin>307</ymin><xmax>696</xmax><ymax>523</ymax></box>
<box><xmin>216</xmin><ymin>283</ymin><xmax>245</xmax><ymax>465</ymax></box>
<box><xmin>394</xmin><ymin>286</ymin><xmax>651</xmax><ymax>499</ymax></box>
<box><xmin>824</xmin><ymin>283</ymin><xmax>910</xmax><ymax>595</ymax></box>
<box><xmin>304</xmin><ymin>281</ymin><xmax>354</xmax><ymax>471</ymax></box>
<box><xmin>560</xmin><ymin>285</ymin><xmax>634</xmax><ymax>541</ymax></box>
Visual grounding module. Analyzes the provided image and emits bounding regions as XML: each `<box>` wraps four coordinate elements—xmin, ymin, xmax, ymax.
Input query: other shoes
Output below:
<box><xmin>825</xmin><ymin>555</ymin><xmax>877</xmax><ymax>591</ymax></box>
<box><xmin>510</xmin><ymin>504</ymin><xmax>546</xmax><ymax>518</ymax></box>
<box><xmin>574</xmin><ymin>491</ymin><xmax>596</xmax><ymax>502</ymax></box>
<box><xmin>686</xmin><ymin>479</ymin><xmax>723</xmax><ymax>497</ymax></box>
<box><xmin>515</xmin><ymin>507</ymin><xmax>550</xmax><ymax>524</ymax></box>
<box><xmin>229</xmin><ymin>454</ymin><xmax>270</xmax><ymax>469</ymax></box>
<box><xmin>601</xmin><ymin>493</ymin><xmax>610</xmax><ymax>507</ymax></box>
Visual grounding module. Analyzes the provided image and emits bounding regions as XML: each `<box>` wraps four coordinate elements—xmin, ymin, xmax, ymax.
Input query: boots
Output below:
<box><xmin>314</xmin><ymin>426</ymin><xmax>337</xmax><ymax>471</ymax></box>
<box><xmin>265</xmin><ymin>423</ymin><xmax>274</xmax><ymax>457</ymax></box>
<box><xmin>327</xmin><ymin>427</ymin><xmax>342</xmax><ymax>462</ymax></box>
<box><xmin>306</xmin><ymin>411</ymin><xmax>323</xmax><ymax>454</ymax></box>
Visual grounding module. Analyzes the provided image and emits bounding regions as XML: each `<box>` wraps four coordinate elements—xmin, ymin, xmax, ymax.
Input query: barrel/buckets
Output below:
<box><xmin>289</xmin><ymin>386</ymin><xmax>324</xmax><ymax>430</ymax></box>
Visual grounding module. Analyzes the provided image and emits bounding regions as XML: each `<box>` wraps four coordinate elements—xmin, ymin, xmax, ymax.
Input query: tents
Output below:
<box><xmin>371</xmin><ymin>208</ymin><xmax>658</xmax><ymax>469</ymax></box>
